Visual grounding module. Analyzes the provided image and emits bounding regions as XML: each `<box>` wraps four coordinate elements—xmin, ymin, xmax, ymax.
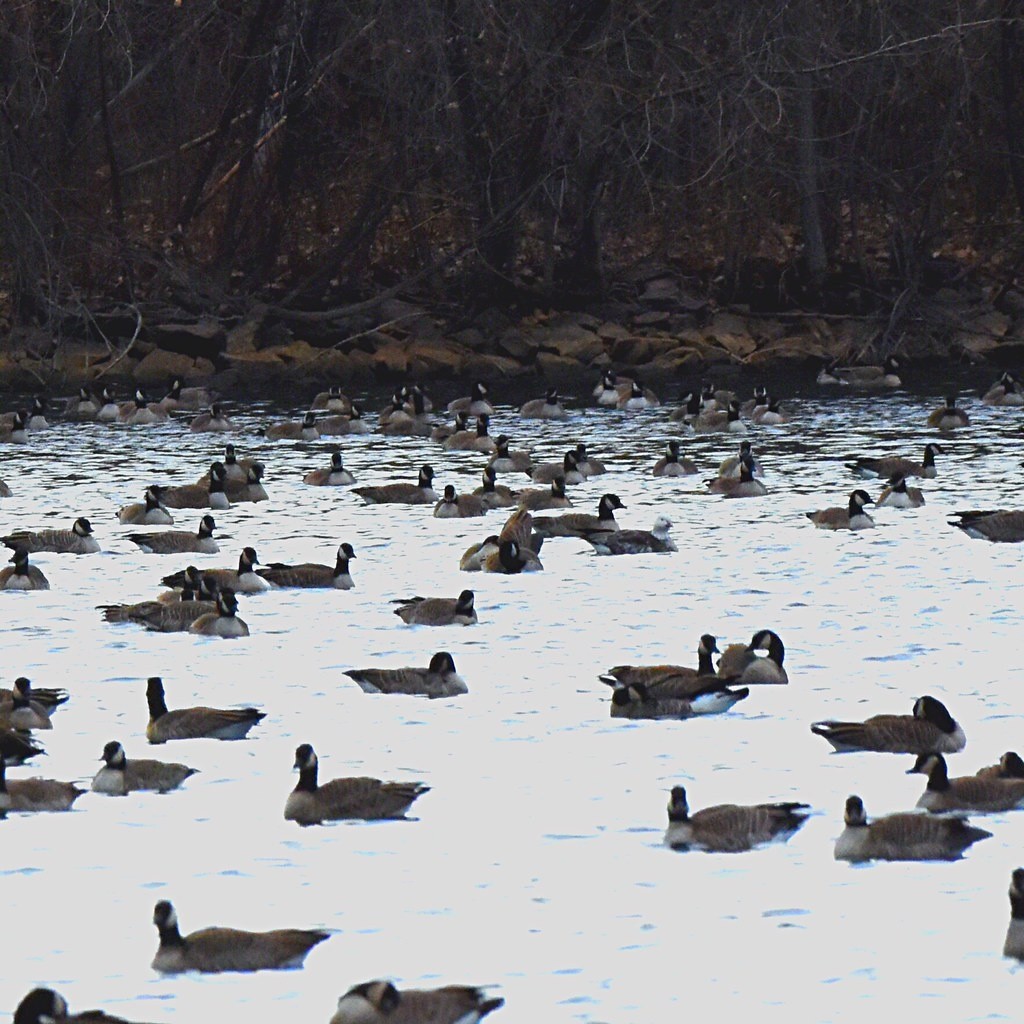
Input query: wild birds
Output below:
<box><xmin>0</xmin><ymin>352</ymin><xmax>1024</xmax><ymax>1024</ymax></box>
